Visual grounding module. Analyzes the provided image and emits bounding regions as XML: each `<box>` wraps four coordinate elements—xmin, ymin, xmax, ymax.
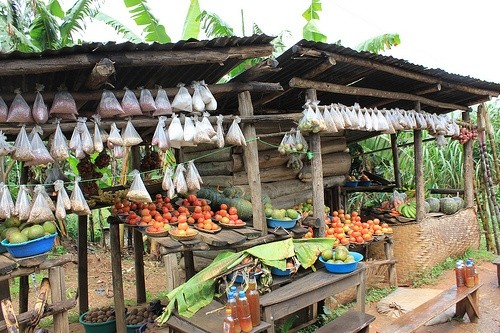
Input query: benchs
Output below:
<box><xmin>378</xmin><ymin>283</ymin><xmax>484</xmax><ymax>333</ymax></box>
<box><xmin>168</xmin><ymin>298</ymin><xmax>271</xmax><ymax>333</ymax></box>
<box><xmin>311</xmin><ymin>309</ymin><xmax>375</xmax><ymax>333</ymax></box>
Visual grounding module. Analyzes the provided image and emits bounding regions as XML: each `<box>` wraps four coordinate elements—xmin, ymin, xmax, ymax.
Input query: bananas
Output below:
<box><xmin>398</xmin><ymin>201</ymin><xmax>417</xmax><ymax>219</ymax></box>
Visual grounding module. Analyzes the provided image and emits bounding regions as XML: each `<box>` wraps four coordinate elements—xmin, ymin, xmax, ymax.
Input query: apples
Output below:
<box><xmin>0</xmin><ymin>217</ymin><xmax>56</xmax><ymax>243</ymax></box>
<box><xmin>278</xmin><ymin>118</ymin><xmax>323</xmax><ymax>155</ymax></box>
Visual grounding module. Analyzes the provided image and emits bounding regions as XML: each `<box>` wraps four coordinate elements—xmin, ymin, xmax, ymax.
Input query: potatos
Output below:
<box><xmin>83</xmin><ymin>306</ymin><xmax>154</xmax><ymax>325</ymax></box>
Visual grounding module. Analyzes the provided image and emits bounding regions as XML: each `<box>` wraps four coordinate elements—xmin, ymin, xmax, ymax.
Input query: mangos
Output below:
<box><xmin>346</xmin><ymin>175</ymin><xmax>368</xmax><ymax>181</ymax></box>
<box><xmin>322</xmin><ymin>245</ymin><xmax>355</xmax><ymax>263</ymax></box>
<box><xmin>265</xmin><ymin>203</ymin><xmax>298</xmax><ymax>220</ymax></box>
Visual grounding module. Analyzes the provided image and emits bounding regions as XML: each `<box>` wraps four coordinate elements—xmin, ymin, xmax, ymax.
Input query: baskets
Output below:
<box><xmin>118</xmin><ymin>198</ymin><xmax>246</xmax><ymax>240</ymax></box>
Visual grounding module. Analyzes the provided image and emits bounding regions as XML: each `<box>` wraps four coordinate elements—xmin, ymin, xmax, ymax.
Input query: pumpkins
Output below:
<box><xmin>424</xmin><ymin>196</ymin><xmax>464</xmax><ymax>215</ymax></box>
<box><xmin>197</xmin><ymin>182</ymin><xmax>271</xmax><ymax>219</ymax></box>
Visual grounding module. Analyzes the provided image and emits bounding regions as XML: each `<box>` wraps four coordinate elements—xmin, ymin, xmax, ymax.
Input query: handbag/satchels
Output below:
<box><xmin>297</xmin><ymin>100</ymin><xmax>460</xmax><ymax>146</ymax></box>
<box><xmin>0</xmin><ymin>176</ymin><xmax>92</xmax><ymax>223</ymax></box>
<box><xmin>277</xmin><ymin>128</ymin><xmax>309</xmax><ymax>170</ymax></box>
<box><xmin>127</xmin><ymin>170</ymin><xmax>152</xmax><ymax>203</ymax></box>
<box><xmin>152</xmin><ymin>111</ymin><xmax>247</xmax><ymax>152</ymax></box>
<box><xmin>0</xmin><ymin>114</ymin><xmax>143</xmax><ymax>167</ymax></box>
<box><xmin>0</xmin><ymin>83</ymin><xmax>79</xmax><ymax>122</ymax></box>
<box><xmin>98</xmin><ymin>81</ymin><xmax>218</xmax><ymax>118</ymax></box>
<box><xmin>162</xmin><ymin>161</ymin><xmax>203</xmax><ymax>199</ymax></box>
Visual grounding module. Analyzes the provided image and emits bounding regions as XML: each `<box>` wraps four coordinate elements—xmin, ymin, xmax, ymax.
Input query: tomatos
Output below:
<box><xmin>111</xmin><ymin>194</ymin><xmax>243</xmax><ymax>235</ymax></box>
<box><xmin>297</xmin><ymin>199</ymin><xmax>392</xmax><ymax>243</ymax></box>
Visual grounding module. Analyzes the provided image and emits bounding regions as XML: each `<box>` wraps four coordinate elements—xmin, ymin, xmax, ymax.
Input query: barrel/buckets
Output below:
<box><xmin>79</xmin><ymin>305</ymin><xmax>120</xmax><ymax>333</ymax></box>
<box><xmin>126</xmin><ymin>324</ymin><xmax>143</xmax><ymax>333</ymax></box>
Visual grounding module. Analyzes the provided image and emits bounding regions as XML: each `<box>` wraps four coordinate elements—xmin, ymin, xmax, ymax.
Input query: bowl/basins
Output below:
<box><xmin>228</xmin><ymin>272</ymin><xmax>262</xmax><ymax>283</ymax></box>
<box><xmin>0</xmin><ymin>231</ymin><xmax>58</xmax><ymax>258</ymax></box>
<box><xmin>267</xmin><ymin>215</ymin><xmax>300</xmax><ymax>229</ymax></box>
<box><xmin>345</xmin><ymin>180</ymin><xmax>374</xmax><ymax>187</ymax></box>
<box><xmin>318</xmin><ymin>251</ymin><xmax>364</xmax><ymax>273</ymax></box>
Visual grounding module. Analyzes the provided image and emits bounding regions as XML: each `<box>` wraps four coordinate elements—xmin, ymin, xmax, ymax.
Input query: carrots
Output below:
<box><xmin>388</xmin><ymin>198</ymin><xmax>404</xmax><ymax>216</ymax></box>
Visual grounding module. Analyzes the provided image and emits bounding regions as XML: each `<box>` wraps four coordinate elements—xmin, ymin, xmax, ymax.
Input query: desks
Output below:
<box><xmin>222</xmin><ymin>262</ymin><xmax>365</xmax><ymax>333</ymax></box>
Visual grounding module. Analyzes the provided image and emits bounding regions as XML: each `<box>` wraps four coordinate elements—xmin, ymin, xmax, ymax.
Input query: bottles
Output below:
<box><xmin>225</xmin><ymin>291</ymin><xmax>242</xmax><ymax>333</ymax></box>
<box><xmin>224</xmin><ymin>309</ymin><xmax>235</xmax><ymax>333</ymax></box>
<box><xmin>229</xmin><ymin>286</ymin><xmax>238</xmax><ymax>301</ymax></box>
<box><xmin>455</xmin><ymin>257</ymin><xmax>478</xmax><ymax>288</ymax></box>
<box><xmin>245</xmin><ymin>282</ymin><xmax>260</xmax><ymax>326</ymax></box>
<box><xmin>237</xmin><ymin>291</ymin><xmax>253</xmax><ymax>332</ymax></box>
